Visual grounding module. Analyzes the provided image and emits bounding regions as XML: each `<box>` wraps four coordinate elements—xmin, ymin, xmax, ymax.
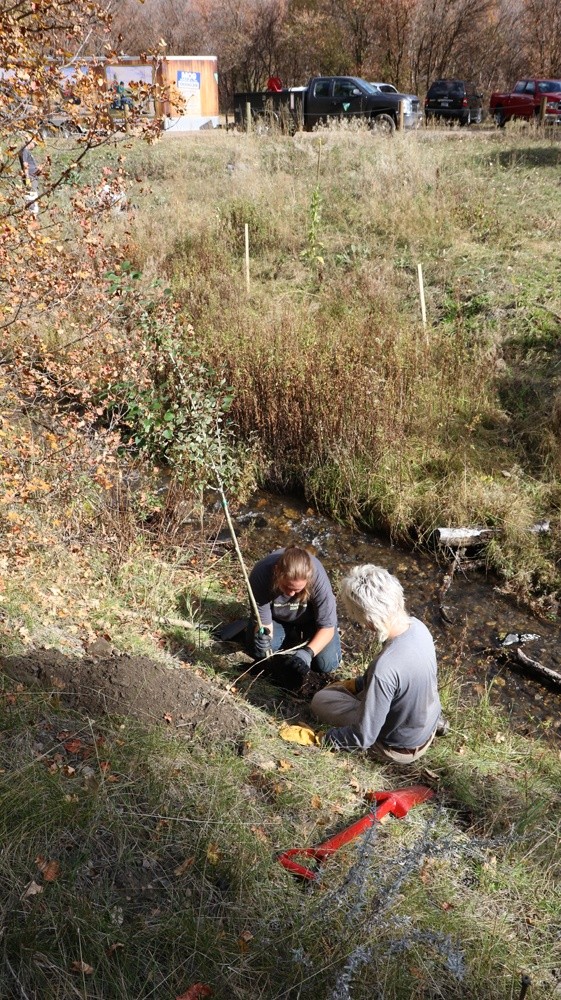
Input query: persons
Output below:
<box><xmin>16</xmin><ymin>140</ymin><xmax>44</xmax><ymax>222</ymax></box>
<box><xmin>244</xmin><ymin>545</ymin><xmax>442</xmax><ymax>770</ymax></box>
<box><xmin>113</xmin><ymin>81</ymin><xmax>129</xmax><ymax>110</ymax></box>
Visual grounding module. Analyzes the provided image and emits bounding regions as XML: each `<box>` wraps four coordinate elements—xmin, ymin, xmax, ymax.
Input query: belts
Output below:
<box><xmin>380</xmin><ymin>729</ymin><xmax>436</xmax><ymax>754</ymax></box>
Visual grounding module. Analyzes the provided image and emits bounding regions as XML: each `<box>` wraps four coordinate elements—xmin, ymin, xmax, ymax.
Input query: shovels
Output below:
<box><xmin>276</xmin><ymin>783</ymin><xmax>437</xmax><ymax>881</ymax></box>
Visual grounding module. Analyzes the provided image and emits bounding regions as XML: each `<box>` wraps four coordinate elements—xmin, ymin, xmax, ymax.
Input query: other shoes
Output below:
<box><xmin>437</xmin><ymin>715</ymin><xmax>450</xmax><ymax>736</ymax></box>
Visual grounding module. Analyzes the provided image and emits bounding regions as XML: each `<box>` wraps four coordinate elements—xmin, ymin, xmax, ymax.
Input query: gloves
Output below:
<box><xmin>253</xmin><ymin>627</ymin><xmax>272</xmax><ymax>651</ymax></box>
<box><xmin>332</xmin><ymin>679</ymin><xmax>357</xmax><ymax>696</ymax></box>
<box><xmin>285</xmin><ymin>647</ymin><xmax>314</xmax><ymax>676</ymax></box>
<box><xmin>280</xmin><ymin>720</ymin><xmax>325</xmax><ymax>747</ymax></box>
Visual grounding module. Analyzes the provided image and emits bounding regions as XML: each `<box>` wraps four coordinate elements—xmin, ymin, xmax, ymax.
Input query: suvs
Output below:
<box><xmin>368</xmin><ymin>81</ymin><xmax>423</xmax><ymax>129</ymax></box>
<box><xmin>424</xmin><ymin>77</ymin><xmax>484</xmax><ymax>127</ymax></box>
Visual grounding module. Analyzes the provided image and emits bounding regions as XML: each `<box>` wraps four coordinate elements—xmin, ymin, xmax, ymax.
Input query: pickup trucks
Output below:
<box><xmin>489</xmin><ymin>78</ymin><xmax>561</xmax><ymax>129</ymax></box>
<box><xmin>233</xmin><ymin>75</ymin><xmax>412</xmax><ymax>137</ymax></box>
